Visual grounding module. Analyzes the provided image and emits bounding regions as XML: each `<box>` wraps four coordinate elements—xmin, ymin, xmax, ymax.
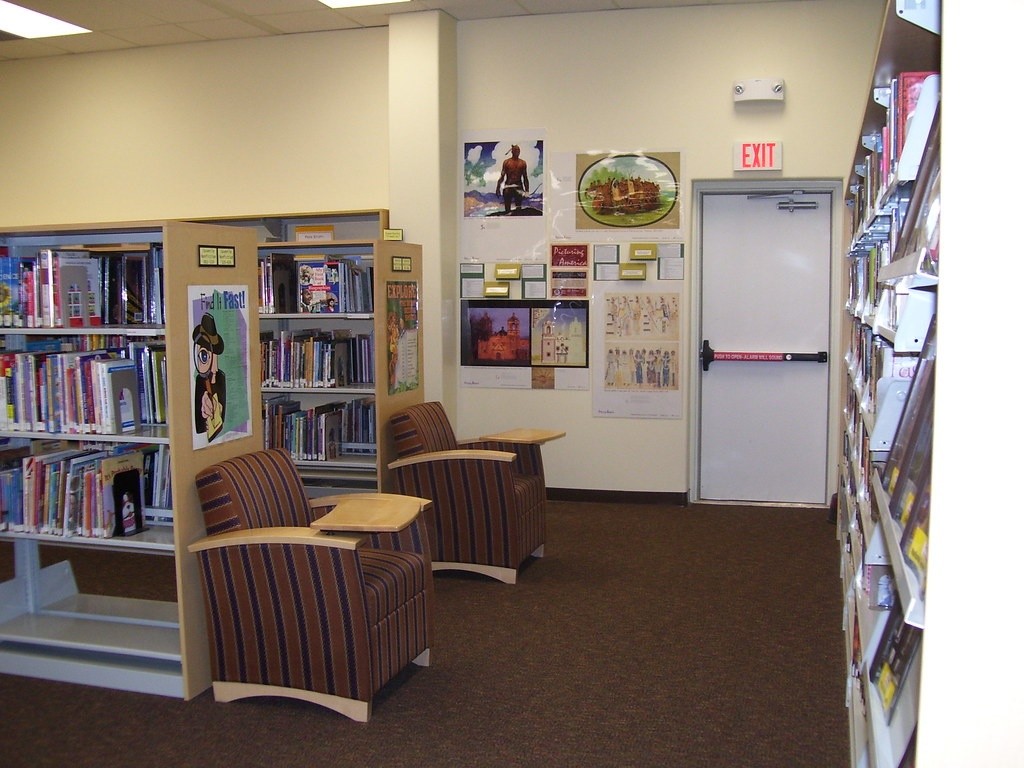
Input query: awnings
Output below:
<box><xmin>260</xmin><ymin>329</ymin><xmax>376</xmax><ymax>389</ymax></box>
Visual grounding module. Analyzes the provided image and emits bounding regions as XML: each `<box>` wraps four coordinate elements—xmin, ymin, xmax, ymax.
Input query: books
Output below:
<box><xmin>0</xmin><ymin>440</ymin><xmax>174</xmax><ymax>539</ymax></box>
<box><xmin>0</xmin><ymin>249</ymin><xmax>164</xmax><ymax>328</ymax></box>
<box><xmin>261</xmin><ymin>396</ymin><xmax>377</xmax><ymax>461</ymax></box>
<box><xmin>258</xmin><ymin>252</ymin><xmax>373</xmax><ymax>314</ymax></box>
<box><xmin>0</xmin><ymin>334</ymin><xmax>168</xmax><ymax>435</ymax></box>
<box><xmin>839</xmin><ymin>71</ymin><xmax>941</xmax><ymax>768</ymax></box>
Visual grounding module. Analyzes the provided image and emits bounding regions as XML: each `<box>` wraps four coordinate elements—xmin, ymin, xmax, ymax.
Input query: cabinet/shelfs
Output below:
<box><xmin>0</xmin><ymin>208</ymin><xmax>389</xmax><ymax>698</ymax></box>
<box><xmin>829</xmin><ymin>0</ymin><xmax>942</xmax><ymax>768</ymax></box>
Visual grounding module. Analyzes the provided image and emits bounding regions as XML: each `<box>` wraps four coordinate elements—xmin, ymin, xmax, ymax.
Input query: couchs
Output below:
<box><xmin>188</xmin><ymin>448</ymin><xmax>433</xmax><ymax>721</ymax></box>
<box><xmin>388</xmin><ymin>401</ymin><xmax>565</xmax><ymax>584</ymax></box>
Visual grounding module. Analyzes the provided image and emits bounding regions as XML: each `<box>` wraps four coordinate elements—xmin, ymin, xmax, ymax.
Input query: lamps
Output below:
<box><xmin>734</xmin><ymin>79</ymin><xmax>784</xmax><ymax>100</ymax></box>
